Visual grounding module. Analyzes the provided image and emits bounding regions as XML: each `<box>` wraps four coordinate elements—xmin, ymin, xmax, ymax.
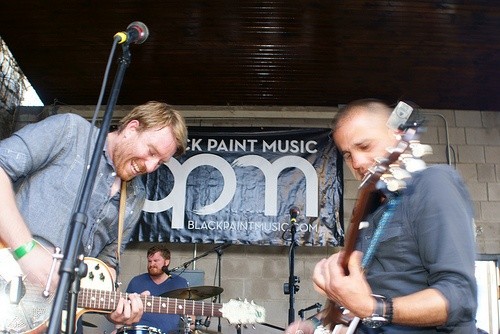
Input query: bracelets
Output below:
<box><xmin>383</xmin><ymin>297</ymin><xmax>393</xmax><ymax>326</ymax></box>
<box><xmin>306</xmin><ymin>316</ymin><xmax>320</xmax><ymax>329</ymax></box>
<box><xmin>12</xmin><ymin>240</ymin><xmax>36</xmax><ymax>260</ymax></box>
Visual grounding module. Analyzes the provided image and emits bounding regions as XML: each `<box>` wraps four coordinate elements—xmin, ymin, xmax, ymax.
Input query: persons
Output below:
<box><xmin>0</xmin><ymin>101</ymin><xmax>189</xmax><ymax>334</ymax></box>
<box><xmin>109</xmin><ymin>244</ymin><xmax>196</xmax><ymax>334</ymax></box>
<box><xmin>288</xmin><ymin>97</ymin><xmax>479</xmax><ymax>334</ymax></box>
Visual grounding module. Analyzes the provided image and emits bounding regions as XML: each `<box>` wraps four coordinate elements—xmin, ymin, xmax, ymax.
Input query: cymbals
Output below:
<box><xmin>158</xmin><ymin>286</ymin><xmax>224</xmax><ymax>301</ymax></box>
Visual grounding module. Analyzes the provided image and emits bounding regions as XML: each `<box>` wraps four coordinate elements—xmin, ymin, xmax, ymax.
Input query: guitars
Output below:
<box><xmin>311</xmin><ymin>127</ymin><xmax>433</xmax><ymax>334</ymax></box>
<box><xmin>0</xmin><ymin>239</ymin><xmax>266</xmax><ymax>334</ymax></box>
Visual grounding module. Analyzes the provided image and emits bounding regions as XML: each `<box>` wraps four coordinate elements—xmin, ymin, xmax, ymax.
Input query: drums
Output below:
<box><xmin>117</xmin><ymin>325</ymin><xmax>166</xmax><ymax>334</ymax></box>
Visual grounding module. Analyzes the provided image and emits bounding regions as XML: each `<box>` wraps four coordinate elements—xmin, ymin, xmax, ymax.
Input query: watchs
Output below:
<box><xmin>362</xmin><ymin>294</ymin><xmax>386</xmax><ymax>329</ymax></box>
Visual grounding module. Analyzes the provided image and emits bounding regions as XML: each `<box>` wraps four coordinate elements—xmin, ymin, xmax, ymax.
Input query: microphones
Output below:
<box><xmin>162</xmin><ymin>266</ymin><xmax>172</xmax><ymax>279</ymax></box>
<box><xmin>289</xmin><ymin>206</ymin><xmax>300</xmax><ymax>224</ymax></box>
<box><xmin>113</xmin><ymin>22</ymin><xmax>149</xmax><ymax>45</ymax></box>
